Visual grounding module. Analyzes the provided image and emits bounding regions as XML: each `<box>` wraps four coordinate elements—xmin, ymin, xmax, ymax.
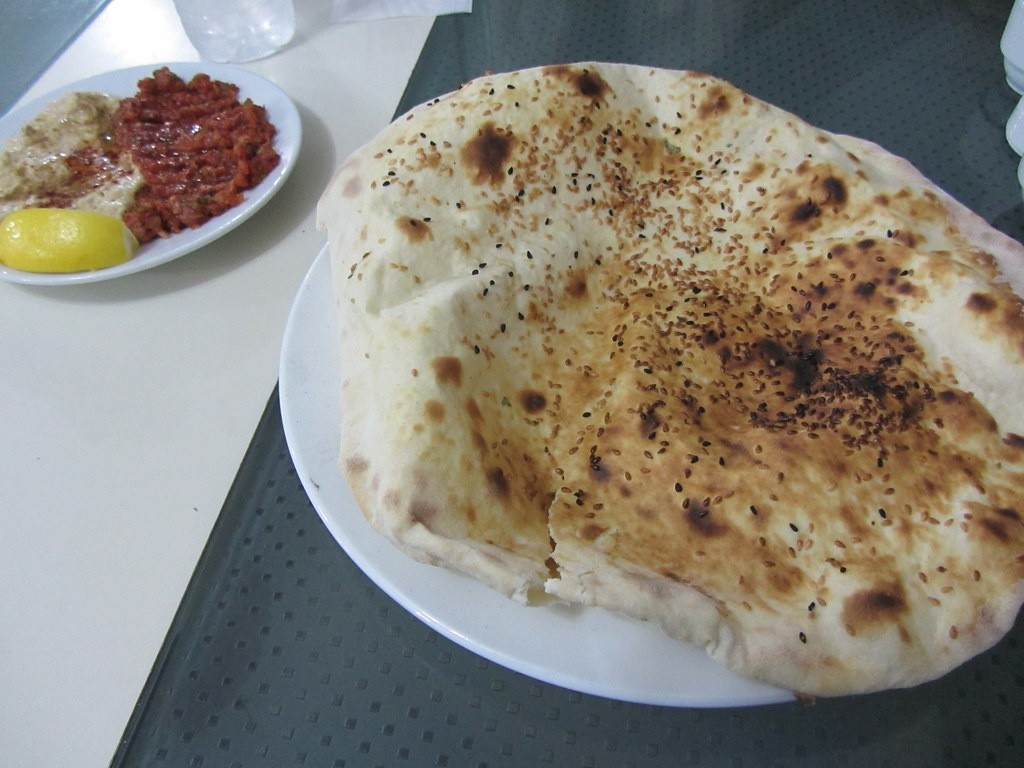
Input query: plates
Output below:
<box><xmin>1</xmin><ymin>61</ymin><xmax>306</xmax><ymax>286</ymax></box>
<box><xmin>277</xmin><ymin>140</ymin><xmax>1023</xmax><ymax>706</ymax></box>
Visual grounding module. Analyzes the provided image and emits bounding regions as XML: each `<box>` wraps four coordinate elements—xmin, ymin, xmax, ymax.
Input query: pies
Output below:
<box><xmin>314</xmin><ymin>61</ymin><xmax>1024</xmax><ymax>698</ymax></box>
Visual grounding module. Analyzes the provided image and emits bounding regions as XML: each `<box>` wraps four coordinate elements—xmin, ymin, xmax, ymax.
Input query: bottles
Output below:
<box><xmin>172</xmin><ymin>0</ymin><xmax>298</xmax><ymax>64</ymax></box>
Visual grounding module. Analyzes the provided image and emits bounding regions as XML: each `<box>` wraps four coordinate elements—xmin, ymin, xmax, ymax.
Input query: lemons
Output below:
<box><xmin>0</xmin><ymin>208</ymin><xmax>139</xmax><ymax>274</ymax></box>
